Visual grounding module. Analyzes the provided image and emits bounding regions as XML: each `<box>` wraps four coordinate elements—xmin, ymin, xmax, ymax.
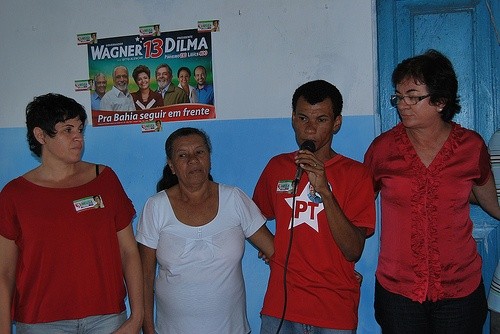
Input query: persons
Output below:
<box><xmin>90</xmin><ymin>72</ymin><xmax>107</xmax><ymax>110</ymax></box>
<box><xmin>87</xmin><ymin>79</ymin><xmax>93</xmax><ymax>90</ymax></box>
<box><xmin>289</xmin><ymin>180</ymin><xmax>296</xmax><ymax>192</ymax></box>
<box><xmin>100</xmin><ymin>66</ymin><xmax>136</xmax><ymax>111</ymax></box>
<box><xmin>259</xmin><ymin>49</ymin><xmax>500</xmax><ymax>334</ymax></box>
<box><xmin>130</xmin><ymin>64</ymin><xmax>164</xmax><ymax>110</ymax></box>
<box><xmin>308</xmin><ymin>184</ymin><xmax>321</xmax><ymax>202</ymax></box>
<box><xmin>154</xmin><ymin>64</ymin><xmax>190</xmax><ymax>106</ymax></box>
<box><xmin>210</xmin><ymin>19</ymin><xmax>220</xmax><ymax>31</ymax></box>
<box><xmin>90</xmin><ymin>32</ymin><xmax>97</xmax><ymax>44</ymax></box>
<box><xmin>93</xmin><ymin>195</ymin><xmax>104</xmax><ymax>208</ymax></box>
<box><xmin>467</xmin><ymin>130</ymin><xmax>500</xmax><ymax>334</ymax></box>
<box><xmin>153</xmin><ymin>24</ymin><xmax>160</xmax><ymax>36</ymax></box>
<box><xmin>154</xmin><ymin>119</ymin><xmax>162</xmax><ymax>131</ymax></box>
<box><xmin>0</xmin><ymin>94</ymin><xmax>143</xmax><ymax>334</ymax></box>
<box><xmin>177</xmin><ymin>66</ymin><xmax>195</xmax><ymax>102</ymax></box>
<box><xmin>252</xmin><ymin>80</ymin><xmax>375</xmax><ymax>334</ymax></box>
<box><xmin>135</xmin><ymin>128</ymin><xmax>363</xmax><ymax>334</ymax></box>
<box><xmin>194</xmin><ymin>65</ymin><xmax>214</xmax><ymax>105</ymax></box>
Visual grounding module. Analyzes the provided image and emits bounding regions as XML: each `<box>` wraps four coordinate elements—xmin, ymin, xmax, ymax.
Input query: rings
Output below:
<box><xmin>313</xmin><ymin>163</ymin><xmax>317</xmax><ymax>168</ymax></box>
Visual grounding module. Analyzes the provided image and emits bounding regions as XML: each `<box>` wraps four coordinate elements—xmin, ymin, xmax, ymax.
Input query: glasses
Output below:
<box><xmin>390</xmin><ymin>93</ymin><xmax>430</xmax><ymax>107</ymax></box>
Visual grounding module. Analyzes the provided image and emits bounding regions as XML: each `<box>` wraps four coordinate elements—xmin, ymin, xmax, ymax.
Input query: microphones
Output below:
<box><xmin>294</xmin><ymin>141</ymin><xmax>315</xmax><ymax>184</ymax></box>
